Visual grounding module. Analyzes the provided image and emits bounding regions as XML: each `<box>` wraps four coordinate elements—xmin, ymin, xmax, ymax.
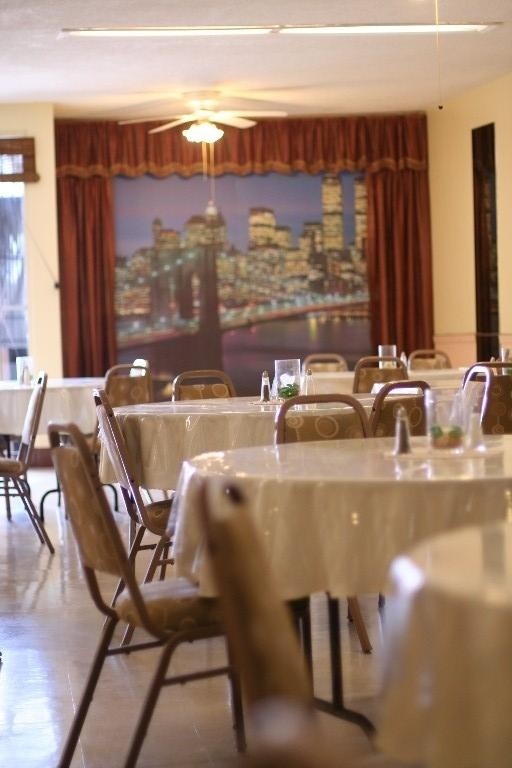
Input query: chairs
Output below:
<box><xmin>463</xmin><ymin>357</ymin><xmax>512</xmax><ymax>436</ymax></box>
<box><xmin>262</xmin><ymin>391</ymin><xmax>374</xmax><ymax>445</ymax></box>
<box><xmin>81</xmin><ymin>361</ymin><xmax>153</xmax><ymax>447</ymax></box>
<box><xmin>349</xmin><ymin>357</ymin><xmax>409</xmax><ymax>395</ymax></box>
<box><xmin>405</xmin><ymin>349</ymin><xmax>448</xmax><ymax>367</ymax></box>
<box><xmin>368</xmin><ymin>376</ymin><xmax>432</xmax><ymax>440</ymax></box>
<box><xmin>169</xmin><ymin>369</ymin><xmax>235</xmax><ymax>400</ymax></box>
<box><xmin>302</xmin><ymin>353</ymin><xmax>346</xmax><ymax>373</ymax></box>
<box><xmin>0</xmin><ymin>372</ymin><xmax>55</xmax><ymax>555</ymax></box>
<box><xmin>82</xmin><ymin>385</ymin><xmax>183</xmax><ymax>651</ymax></box>
<box><xmin>123</xmin><ymin>356</ymin><xmax>150</xmax><ymax>376</ymax></box>
<box><xmin>191</xmin><ymin>479</ymin><xmax>380</xmax><ymax>768</ymax></box>
<box><xmin>42</xmin><ymin>417</ymin><xmax>246</xmax><ymax>768</ymax></box>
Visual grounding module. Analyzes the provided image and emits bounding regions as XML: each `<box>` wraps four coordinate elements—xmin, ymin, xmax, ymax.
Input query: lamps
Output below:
<box><xmin>181</xmin><ymin>110</ymin><xmax>225</xmax><ymax>147</ymax></box>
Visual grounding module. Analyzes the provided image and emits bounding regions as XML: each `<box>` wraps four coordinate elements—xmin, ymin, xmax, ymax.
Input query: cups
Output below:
<box><xmin>274</xmin><ymin>358</ymin><xmax>301</xmax><ymax>402</ymax></box>
<box><xmin>424</xmin><ymin>387</ymin><xmax>472</xmax><ymax>456</ymax></box>
<box><xmin>378</xmin><ymin>344</ymin><xmax>397</xmax><ymax>371</ymax></box>
<box><xmin>15</xmin><ymin>356</ymin><xmax>34</xmax><ymax>386</ymax></box>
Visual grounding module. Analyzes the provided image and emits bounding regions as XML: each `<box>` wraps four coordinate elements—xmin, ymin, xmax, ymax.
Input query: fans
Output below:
<box><xmin>113</xmin><ymin>101</ymin><xmax>290</xmax><ymax>135</ymax></box>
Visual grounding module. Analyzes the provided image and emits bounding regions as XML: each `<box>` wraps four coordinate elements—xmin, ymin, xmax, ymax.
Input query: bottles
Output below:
<box><xmin>394</xmin><ymin>406</ymin><xmax>412</xmax><ymax>456</ymax></box>
<box><xmin>466</xmin><ymin>403</ymin><xmax>481</xmax><ymax>450</ymax></box>
<box><xmin>257</xmin><ymin>369</ymin><xmax>270</xmax><ymax>402</ymax></box>
<box><xmin>303</xmin><ymin>368</ymin><xmax>315</xmax><ymax>403</ymax></box>
